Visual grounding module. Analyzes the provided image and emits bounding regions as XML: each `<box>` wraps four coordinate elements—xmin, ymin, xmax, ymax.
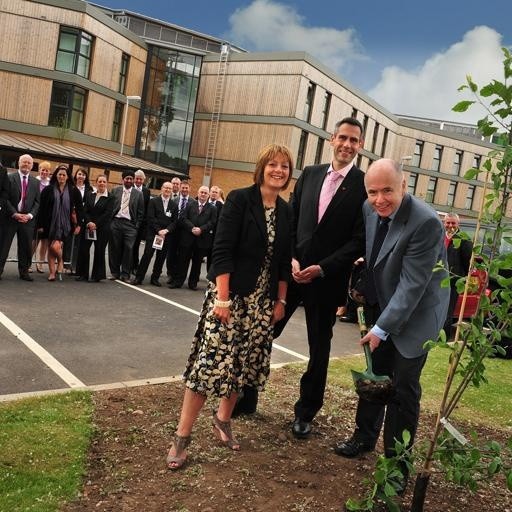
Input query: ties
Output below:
<box><xmin>199</xmin><ymin>203</ymin><xmax>204</xmax><ymax>215</ymax></box>
<box><xmin>370</xmin><ymin>214</ymin><xmax>394</xmax><ymax>274</ymax></box>
<box><xmin>164</xmin><ymin>200</ymin><xmax>168</xmax><ymax>213</ymax></box>
<box><xmin>179</xmin><ymin>198</ymin><xmax>187</xmax><ymax>218</ymax></box>
<box><xmin>21</xmin><ymin>175</ymin><xmax>28</xmax><ymax>201</ymax></box>
<box><xmin>122</xmin><ymin>190</ymin><xmax>131</xmax><ymax>215</ymax></box>
<box><xmin>317</xmin><ymin>169</ymin><xmax>342</xmax><ymax>225</ymax></box>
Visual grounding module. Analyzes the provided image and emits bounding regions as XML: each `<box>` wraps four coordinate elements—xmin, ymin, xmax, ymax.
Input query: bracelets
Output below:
<box><xmin>276</xmin><ymin>297</ymin><xmax>287</xmax><ymax>305</ymax></box>
<box><xmin>320</xmin><ymin>267</ymin><xmax>326</xmax><ymax>279</ymax></box>
<box><xmin>213</xmin><ymin>298</ymin><xmax>233</xmax><ymax>309</ymax></box>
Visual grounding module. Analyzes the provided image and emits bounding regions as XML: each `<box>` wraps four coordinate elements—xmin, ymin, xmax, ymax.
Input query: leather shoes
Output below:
<box><xmin>212</xmin><ymin>399</ymin><xmax>260</xmax><ymax>417</ymax></box>
<box><xmin>292</xmin><ymin>412</ymin><xmax>312</xmax><ymax>439</ymax></box>
<box><xmin>18</xmin><ymin>262</ymin><xmax>202</xmax><ymax>292</ymax></box>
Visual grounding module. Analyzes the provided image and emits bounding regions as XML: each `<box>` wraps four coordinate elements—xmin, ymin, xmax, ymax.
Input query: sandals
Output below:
<box><xmin>210</xmin><ymin>415</ymin><xmax>241</xmax><ymax>452</ymax></box>
<box><xmin>165</xmin><ymin>431</ymin><xmax>192</xmax><ymax>472</ymax></box>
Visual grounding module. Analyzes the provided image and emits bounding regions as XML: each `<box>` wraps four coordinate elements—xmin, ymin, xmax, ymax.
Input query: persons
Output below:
<box><xmin>336</xmin><ymin>212</ymin><xmax>474</xmax><ymax>336</ymax></box>
<box><xmin>333</xmin><ymin>157</ymin><xmax>452</xmax><ymax>497</ymax></box>
<box><xmin>129</xmin><ymin>178</ymin><xmax>226</xmax><ymax>290</ymax></box>
<box><xmin>0</xmin><ymin>155</ymin><xmax>149</xmax><ymax>282</ymax></box>
<box><xmin>163</xmin><ymin>143</ymin><xmax>296</xmax><ymax>470</ymax></box>
<box><xmin>214</xmin><ymin>117</ymin><xmax>370</xmax><ymax>440</ymax></box>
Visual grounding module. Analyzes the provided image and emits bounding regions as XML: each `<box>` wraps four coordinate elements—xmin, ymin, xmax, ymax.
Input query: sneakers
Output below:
<box><xmin>387</xmin><ymin>469</ymin><xmax>411</xmax><ymax>500</ymax></box>
<box><xmin>335</xmin><ymin>437</ymin><xmax>377</xmax><ymax>456</ymax></box>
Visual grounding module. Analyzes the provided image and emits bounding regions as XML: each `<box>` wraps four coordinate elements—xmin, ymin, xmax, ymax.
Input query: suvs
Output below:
<box><xmin>436</xmin><ymin>214</ymin><xmax>512</xmax><ymax>323</ymax></box>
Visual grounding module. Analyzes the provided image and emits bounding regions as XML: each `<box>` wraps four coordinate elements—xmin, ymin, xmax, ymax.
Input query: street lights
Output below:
<box><xmin>120</xmin><ymin>94</ymin><xmax>142</xmax><ymax>157</ymax></box>
<box><xmin>401</xmin><ymin>156</ymin><xmax>413</xmax><ymax>168</ymax></box>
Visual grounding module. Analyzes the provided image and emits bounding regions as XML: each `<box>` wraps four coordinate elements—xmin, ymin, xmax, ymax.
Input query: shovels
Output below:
<box><xmin>351</xmin><ymin>292</ymin><xmax>393</xmax><ymax>405</ymax></box>
